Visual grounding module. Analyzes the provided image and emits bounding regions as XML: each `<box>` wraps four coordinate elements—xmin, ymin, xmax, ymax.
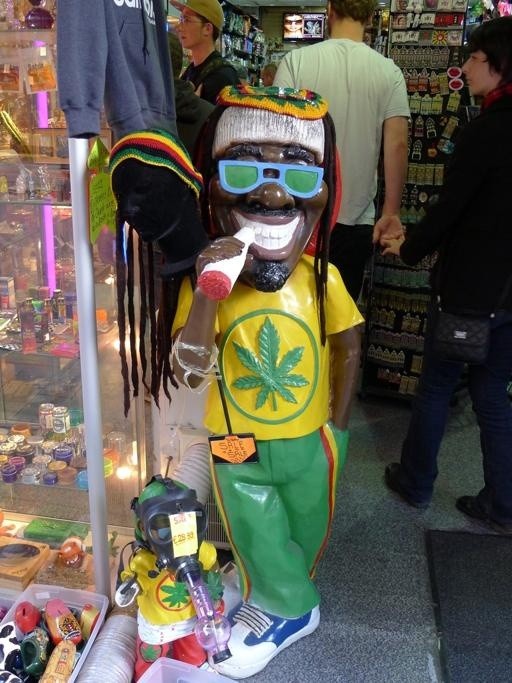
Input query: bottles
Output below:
<box><xmin>223</xmin><ymin>10</ymin><xmax>265</xmax><ymax>72</ymax></box>
<box><xmin>38</xmin><ymin>403</ymin><xmax>56</xmax><ymax>438</ymax></box>
<box><xmin>51</xmin><ymin>405</ymin><xmax>73</xmax><ymax>441</ymax></box>
<box><xmin>25</xmin><ymin>285</ymin><xmax>66</xmax><ymax>328</ymax></box>
<box><xmin>196</xmin><ymin>224</ymin><xmax>258</xmax><ymax>300</ymax></box>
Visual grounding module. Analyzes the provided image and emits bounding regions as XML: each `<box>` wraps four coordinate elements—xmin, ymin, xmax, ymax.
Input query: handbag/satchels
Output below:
<box><xmin>428</xmin><ymin>310</ymin><xmax>495</xmax><ymax>362</ymax></box>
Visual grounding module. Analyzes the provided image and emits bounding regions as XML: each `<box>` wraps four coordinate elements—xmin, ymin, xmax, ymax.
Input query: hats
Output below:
<box><xmin>170</xmin><ymin>0</ymin><xmax>225</xmax><ymax>32</ymax></box>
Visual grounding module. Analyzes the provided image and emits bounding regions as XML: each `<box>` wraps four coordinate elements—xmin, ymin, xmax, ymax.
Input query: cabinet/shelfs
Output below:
<box><xmin>164</xmin><ymin>0</ymin><xmax>264</xmax><ymax>88</ymax></box>
<box><xmin>0</xmin><ymin>0</ymin><xmax>157</xmax><ymax>603</ymax></box>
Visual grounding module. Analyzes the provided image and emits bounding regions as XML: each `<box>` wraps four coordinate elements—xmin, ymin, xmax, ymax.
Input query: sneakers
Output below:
<box><xmin>456</xmin><ymin>495</ymin><xmax>512</xmax><ymax>532</ymax></box>
<box><xmin>385</xmin><ymin>461</ymin><xmax>432</xmax><ymax>510</ymax></box>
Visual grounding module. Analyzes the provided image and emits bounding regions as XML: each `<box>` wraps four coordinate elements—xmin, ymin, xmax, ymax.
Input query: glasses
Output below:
<box><xmin>284</xmin><ymin>19</ymin><xmax>303</xmax><ymax>26</ymax></box>
<box><xmin>178</xmin><ymin>14</ymin><xmax>204</xmax><ymax>26</ymax></box>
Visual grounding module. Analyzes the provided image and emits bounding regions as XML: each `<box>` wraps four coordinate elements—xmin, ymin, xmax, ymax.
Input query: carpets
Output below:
<box><xmin>425</xmin><ymin>528</ymin><xmax>512</xmax><ymax>683</ymax></box>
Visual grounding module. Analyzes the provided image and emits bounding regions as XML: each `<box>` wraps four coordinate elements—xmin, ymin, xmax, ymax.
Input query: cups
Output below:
<box><xmin>0</xmin><ymin>421</ymin><xmax>130</xmax><ymax>491</ymax></box>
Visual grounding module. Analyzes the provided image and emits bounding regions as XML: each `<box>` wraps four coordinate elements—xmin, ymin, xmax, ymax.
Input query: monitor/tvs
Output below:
<box><xmin>282</xmin><ymin>13</ymin><xmax>325</xmax><ymax>41</ymax></box>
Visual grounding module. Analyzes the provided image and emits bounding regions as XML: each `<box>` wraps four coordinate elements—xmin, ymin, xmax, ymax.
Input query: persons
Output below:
<box><xmin>115</xmin><ymin>471</ymin><xmax>226</xmax><ymax>682</ymax></box>
<box><xmin>259</xmin><ymin>62</ymin><xmax>276</xmax><ymax>86</ymax></box>
<box><xmin>168</xmin><ymin>0</ymin><xmax>240</xmax><ymax>102</ymax></box>
<box><xmin>283</xmin><ymin>12</ymin><xmax>303</xmax><ymax>37</ymax></box>
<box><xmin>108</xmin><ymin>126</ymin><xmax>214</xmax><ymax>418</ymax></box>
<box><xmin>303</xmin><ymin>19</ymin><xmax>321</xmax><ymax>34</ymax></box>
<box><xmin>166</xmin><ymin>30</ymin><xmax>216</xmax><ymax>154</ymax></box>
<box><xmin>162</xmin><ymin>78</ymin><xmax>364</xmax><ymax>676</ymax></box>
<box><xmin>379</xmin><ymin>11</ymin><xmax>512</xmax><ymax>524</ymax></box>
<box><xmin>272</xmin><ymin>0</ymin><xmax>416</xmax><ymax>308</ymax></box>
